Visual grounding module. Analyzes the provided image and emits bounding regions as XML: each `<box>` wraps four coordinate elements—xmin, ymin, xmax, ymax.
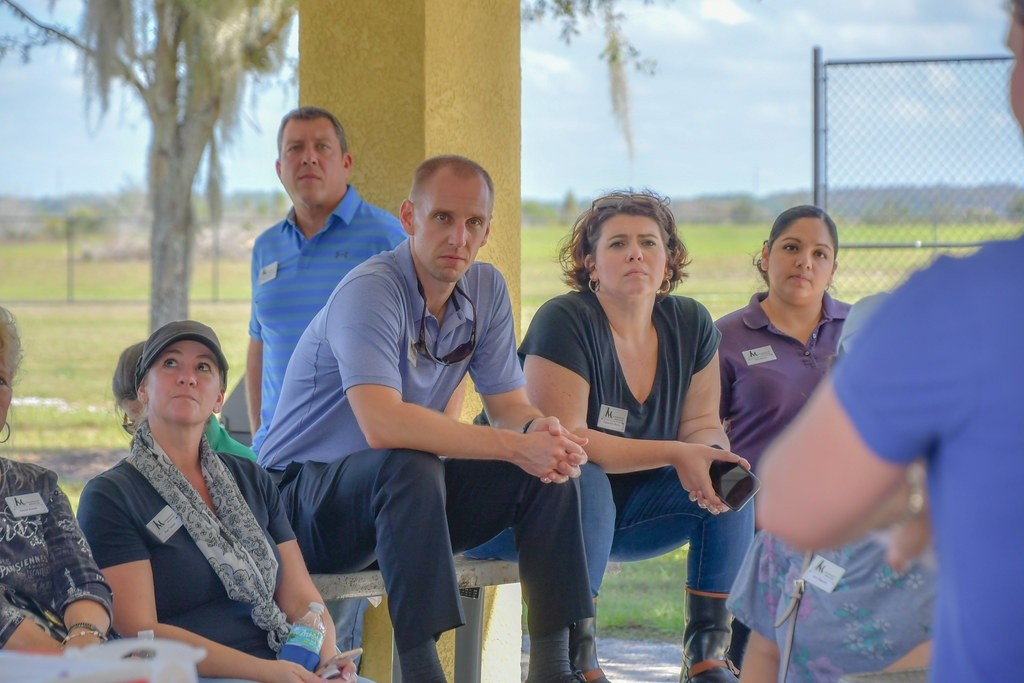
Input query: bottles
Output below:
<box><xmin>279</xmin><ymin>601</ymin><xmax>327</xmax><ymax>674</ymax></box>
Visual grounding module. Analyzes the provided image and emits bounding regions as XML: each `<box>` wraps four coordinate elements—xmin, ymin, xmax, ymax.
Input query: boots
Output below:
<box><xmin>569</xmin><ymin>598</ymin><xmax>612</xmax><ymax>683</ymax></box>
<box><xmin>678</xmin><ymin>586</ymin><xmax>740</xmax><ymax>683</ymax></box>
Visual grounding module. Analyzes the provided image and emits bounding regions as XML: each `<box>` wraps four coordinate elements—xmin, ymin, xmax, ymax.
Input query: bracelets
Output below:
<box><xmin>522</xmin><ymin>418</ymin><xmax>534</xmax><ymax>433</ymax></box>
<box><xmin>59</xmin><ymin>630</ymin><xmax>108</xmax><ymax>650</ymax></box>
<box><xmin>66</xmin><ymin>622</ymin><xmax>104</xmax><ymax>636</ymax></box>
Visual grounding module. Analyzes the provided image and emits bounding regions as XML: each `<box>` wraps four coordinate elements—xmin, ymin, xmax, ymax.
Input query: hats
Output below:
<box><xmin>133</xmin><ymin>319</ymin><xmax>229</xmax><ymax>393</ymax></box>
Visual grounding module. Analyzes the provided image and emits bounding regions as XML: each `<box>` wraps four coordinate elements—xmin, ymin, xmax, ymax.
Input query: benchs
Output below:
<box><xmin>310</xmin><ymin>557</ymin><xmax>522</xmax><ymax>683</ymax></box>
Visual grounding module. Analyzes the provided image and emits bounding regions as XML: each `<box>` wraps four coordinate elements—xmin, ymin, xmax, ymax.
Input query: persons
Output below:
<box><xmin>0</xmin><ymin>309</ymin><xmax>114</xmax><ymax>656</ymax></box>
<box><xmin>463</xmin><ymin>189</ymin><xmax>757</xmax><ymax>683</ymax></box>
<box><xmin>245</xmin><ymin>107</ymin><xmax>407</xmax><ymax>675</ymax></box>
<box><xmin>725</xmin><ymin>527</ymin><xmax>933</xmax><ymax>683</ymax></box>
<box><xmin>714</xmin><ymin>204</ymin><xmax>852</xmax><ymax>475</ymax></box>
<box><xmin>112</xmin><ymin>342</ymin><xmax>257</xmax><ymax>463</ymax></box>
<box><xmin>758</xmin><ymin>0</ymin><xmax>1023</xmax><ymax>680</ymax></box>
<box><xmin>75</xmin><ymin>320</ymin><xmax>376</xmax><ymax>683</ymax></box>
<box><xmin>257</xmin><ymin>156</ymin><xmax>596</xmax><ymax>683</ymax></box>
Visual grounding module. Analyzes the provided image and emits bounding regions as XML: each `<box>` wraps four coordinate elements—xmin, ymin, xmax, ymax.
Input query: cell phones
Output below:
<box><xmin>315</xmin><ymin>648</ymin><xmax>363</xmax><ymax>675</ymax></box>
<box><xmin>709</xmin><ymin>444</ymin><xmax>762</xmax><ymax>512</ymax></box>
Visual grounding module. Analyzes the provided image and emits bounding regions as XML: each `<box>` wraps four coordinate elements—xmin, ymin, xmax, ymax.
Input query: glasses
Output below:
<box><xmin>413</xmin><ymin>279</ymin><xmax>476</xmax><ymax>366</ymax></box>
<box><xmin>591</xmin><ymin>195</ymin><xmax>662</xmax><ymax>211</ymax></box>
<box><xmin>122</xmin><ymin>413</ymin><xmax>138</xmax><ymax>435</ymax></box>
